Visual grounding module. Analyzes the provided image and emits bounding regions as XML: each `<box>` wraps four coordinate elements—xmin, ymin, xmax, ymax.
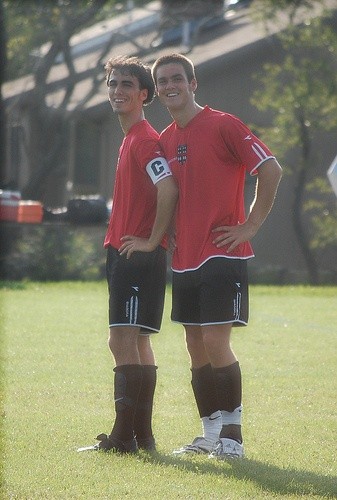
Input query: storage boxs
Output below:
<box><xmin>0</xmin><ymin>199</ymin><xmax>44</xmax><ymax>225</ymax></box>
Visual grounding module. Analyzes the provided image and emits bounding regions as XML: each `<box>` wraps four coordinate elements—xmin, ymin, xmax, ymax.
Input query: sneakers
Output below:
<box><xmin>134</xmin><ymin>437</ymin><xmax>156</xmax><ymax>453</ymax></box>
<box><xmin>168</xmin><ymin>436</ymin><xmax>219</xmax><ymax>456</ymax></box>
<box><xmin>76</xmin><ymin>432</ymin><xmax>140</xmax><ymax>457</ymax></box>
<box><xmin>205</xmin><ymin>438</ymin><xmax>245</xmax><ymax>460</ymax></box>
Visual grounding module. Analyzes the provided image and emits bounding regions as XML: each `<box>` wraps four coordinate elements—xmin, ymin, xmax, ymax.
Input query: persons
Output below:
<box><xmin>77</xmin><ymin>55</ymin><xmax>176</xmax><ymax>452</ymax></box>
<box><xmin>152</xmin><ymin>55</ymin><xmax>284</xmax><ymax>459</ymax></box>
